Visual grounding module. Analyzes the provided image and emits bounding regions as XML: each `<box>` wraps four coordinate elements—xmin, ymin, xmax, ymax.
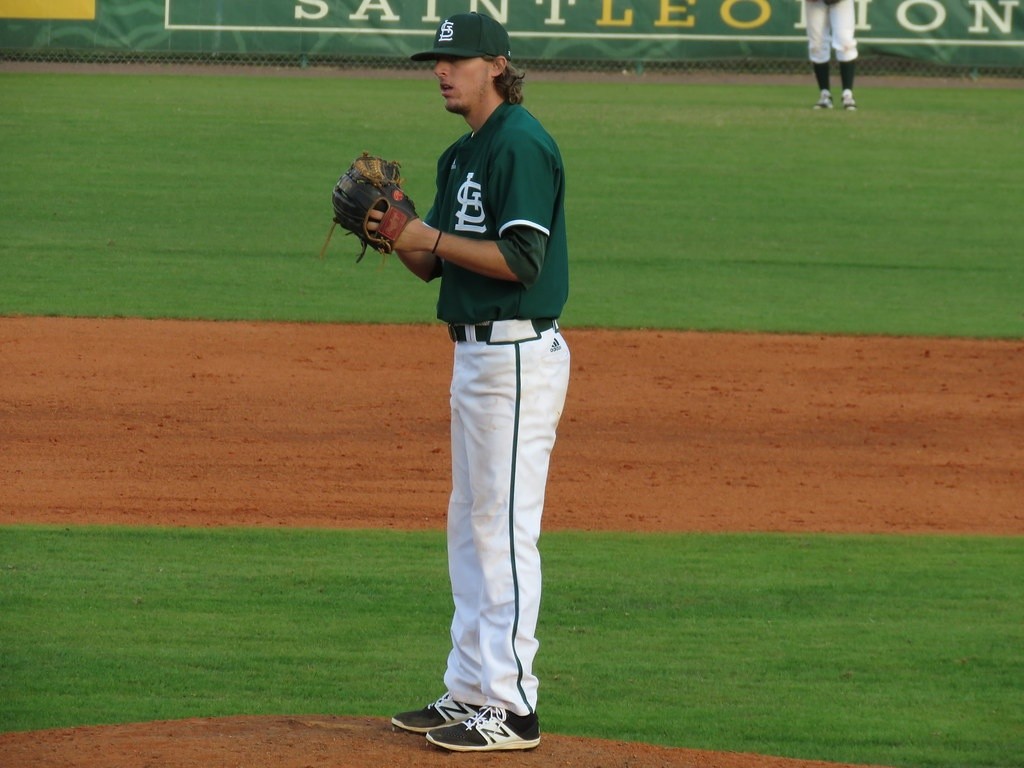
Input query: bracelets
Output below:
<box><xmin>431</xmin><ymin>230</ymin><xmax>443</xmax><ymax>255</ymax></box>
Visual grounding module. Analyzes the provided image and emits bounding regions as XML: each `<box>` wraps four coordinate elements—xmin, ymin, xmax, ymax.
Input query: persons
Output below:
<box><xmin>803</xmin><ymin>0</ymin><xmax>860</xmax><ymax>111</ymax></box>
<box><xmin>329</xmin><ymin>13</ymin><xmax>574</xmax><ymax>752</ymax></box>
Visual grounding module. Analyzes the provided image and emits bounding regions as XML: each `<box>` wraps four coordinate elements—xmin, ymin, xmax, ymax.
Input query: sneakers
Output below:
<box><xmin>391</xmin><ymin>692</ymin><xmax>483</xmax><ymax>732</ymax></box>
<box><xmin>813</xmin><ymin>90</ymin><xmax>833</xmax><ymax>109</ymax></box>
<box><xmin>426</xmin><ymin>706</ymin><xmax>541</xmax><ymax>752</ymax></box>
<box><xmin>841</xmin><ymin>90</ymin><xmax>856</xmax><ymax>110</ymax></box>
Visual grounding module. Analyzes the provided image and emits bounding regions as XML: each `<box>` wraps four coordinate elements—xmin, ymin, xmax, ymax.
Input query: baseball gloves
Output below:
<box><xmin>330</xmin><ymin>151</ymin><xmax>420</xmax><ymax>255</ymax></box>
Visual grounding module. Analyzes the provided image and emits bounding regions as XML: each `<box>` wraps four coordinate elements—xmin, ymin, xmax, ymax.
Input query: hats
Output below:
<box><xmin>410</xmin><ymin>10</ymin><xmax>511</xmax><ymax>60</ymax></box>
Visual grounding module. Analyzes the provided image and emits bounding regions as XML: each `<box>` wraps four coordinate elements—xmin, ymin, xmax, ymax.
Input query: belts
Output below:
<box><xmin>448</xmin><ymin>317</ymin><xmax>553</xmax><ymax>343</ymax></box>
